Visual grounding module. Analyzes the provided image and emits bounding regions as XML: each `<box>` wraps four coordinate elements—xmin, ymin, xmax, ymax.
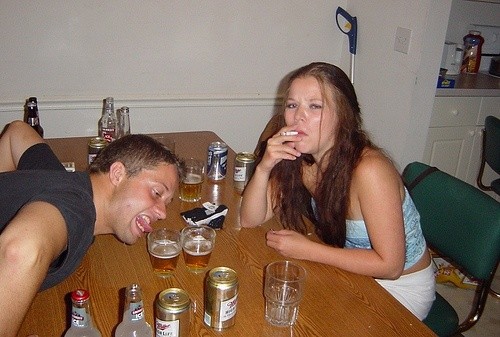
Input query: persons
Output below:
<box><xmin>239</xmin><ymin>62</ymin><xmax>434</xmax><ymax>321</ymax></box>
<box><xmin>0</xmin><ymin>121</ymin><xmax>187</xmax><ymax>337</ymax></box>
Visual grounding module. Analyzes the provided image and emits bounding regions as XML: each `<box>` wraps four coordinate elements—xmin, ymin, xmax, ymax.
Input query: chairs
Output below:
<box><xmin>476</xmin><ymin>115</ymin><xmax>500</xmax><ymax>196</ymax></box>
<box><xmin>400</xmin><ymin>162</ymin><xmax>500</xmax><ymax>337</ymax></box>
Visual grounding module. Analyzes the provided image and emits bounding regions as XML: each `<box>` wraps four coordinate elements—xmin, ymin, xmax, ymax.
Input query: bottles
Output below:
<box><xmin>463</xmin><ymin>30</ymin><xmax>484</xmax><ymax>75</ymax></box>
<box><xmin>27</xmin><ymin>97</ymin><xmax>44</xmax><ymax>138</ymax></box>
<box><xmin>99</xmin><ymin>97</ymin><xmax>130</xmax><ymax>142</ymax></box>
<box><xmin>115</xmin><ymin>284</ymin><xmax>152</xmax><ymax>337</ymax></box>
<box><xmin>64</xmin><ymin>289</ymin><xmax>101</xmax><ymax>337</ymax></box>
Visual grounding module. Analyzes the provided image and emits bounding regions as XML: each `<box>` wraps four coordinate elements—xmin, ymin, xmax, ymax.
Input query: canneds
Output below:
<box><xmin>156</xmin><ymin>287</ymin><xmax>192</xmax><ymax>337</ymax></box>
<box><xmin>204</xmin><ymin>266</ymin><xmax>239</xmax><ymax>332</ymax></box>
<box><xmin>232</xmin><ymin>152</ymin><xmax>257</xmax><ymax>195</ymax></box>
<box><xmin>207</xmin><ymin>141</ymin><xmax>228</xmax><ymax>182</ymax></box>
<box><xmin>88</xmin><ymin>137</ymin><xmax>109</xmax><ymax>165</ymax></box>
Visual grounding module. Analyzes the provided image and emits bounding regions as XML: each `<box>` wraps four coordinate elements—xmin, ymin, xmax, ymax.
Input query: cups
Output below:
<box><xmin>178</xmin><ymin>156</ymin><xmax>204</xmax><ymax>202</ymax></box>
<box><xmin>146</xmin><ymin>226</ymin><xmax>184</xmax><ymax>278</ymax></box>
<box><xmin>153</xmin><ymin>137</ymin><xmax>175</xmax><ymax>154</ymax></box>
<box><xmin>181</xmin><ymin>225</ymin><xmax>216</xmax><ymax>272</ymax></box>
<box><xmin>61</xmin><ymin>162</ymin><xmax>74</xmax><ymax>172</ymax></box>
<box><xmin>264</xmin><ymin>261</ymin><xmax>306</xmax><ymax>327</ymax></box>
<box><xmin>441</xmin><ymin>43</ymin><xmax>463</xmax><ymax>73</ymax></box>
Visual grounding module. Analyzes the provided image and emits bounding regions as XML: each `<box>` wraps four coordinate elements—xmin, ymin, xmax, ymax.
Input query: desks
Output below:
<box><xmin>17</xmin><ymin>131</ymin><xmax>439</xmax><ymax>337</ymax></box>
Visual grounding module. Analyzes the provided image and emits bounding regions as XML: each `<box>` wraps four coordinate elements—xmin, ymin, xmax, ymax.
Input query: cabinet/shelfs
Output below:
<box><xmin>425</xmin><ymin>97</ymin><xmax>500</xmax><ymax>202</ymax></box>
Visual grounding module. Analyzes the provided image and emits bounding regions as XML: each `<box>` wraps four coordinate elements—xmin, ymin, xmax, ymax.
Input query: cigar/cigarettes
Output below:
<box><xmin>281</xmin><ymin>131</ymin><xmax>298</xmax><ymax>136</ymax></box>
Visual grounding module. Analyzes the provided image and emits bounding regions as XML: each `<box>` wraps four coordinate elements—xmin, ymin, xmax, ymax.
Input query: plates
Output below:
<box><xmin>446</xmin><ymin>73</ymin><xmax>461</xmax><ymax>75</ymax></box>
